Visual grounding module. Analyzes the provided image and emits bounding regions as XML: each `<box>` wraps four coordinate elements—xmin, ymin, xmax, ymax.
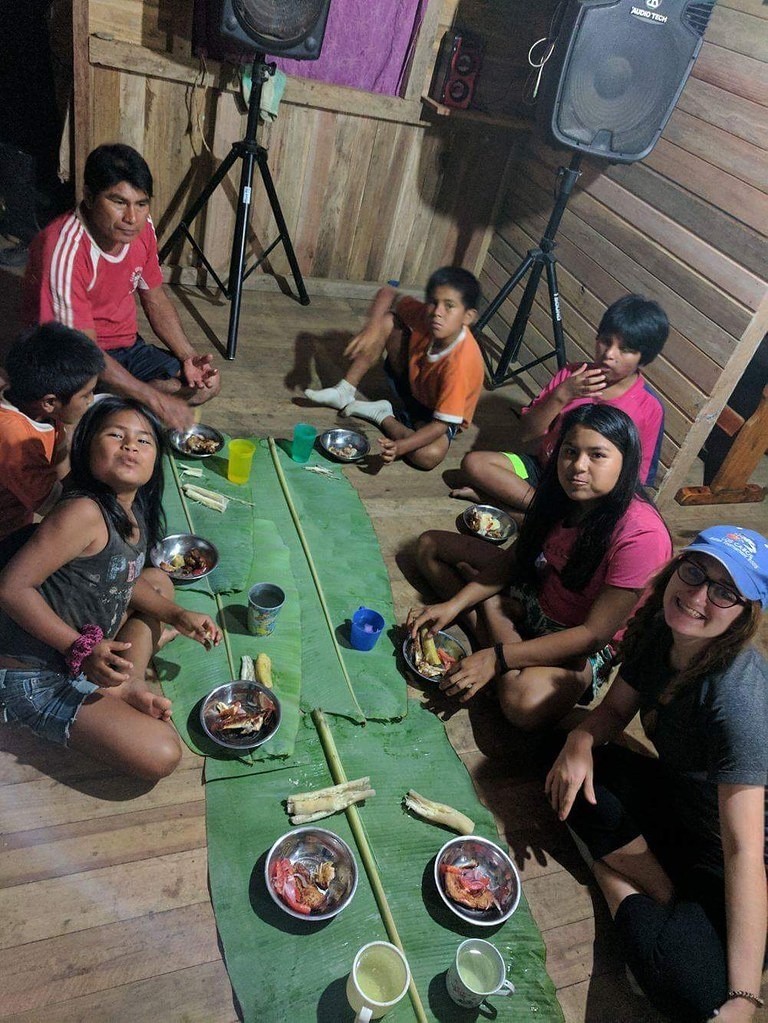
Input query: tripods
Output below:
<box><xmin>472</xmin><ymin>151</ymin><xmax>586</xmax><ymax>392</ymax></box>
<box><xmin>159</xmin><ymin>52</ymin><xmax>310</xmax><ymax>360</ymax></box>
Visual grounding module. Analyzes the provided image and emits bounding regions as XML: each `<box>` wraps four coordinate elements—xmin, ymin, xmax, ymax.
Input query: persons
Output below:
<box><xmin>545</xmin><ymin>523</ymin><xmax>768</xmax><ymax>1023</ymax></box>
<box><xmin>405</xmin><ymin>397</ymin><xmax>674</xmax><ymax>728</ymax></box>
<box><xmin>0</xmin><ymin>0</ymin><xmax>63</xmax><ymax>263</ymax></box>
<box><xmin>0</xmin><ymin>395</ymin><xmax>224</xmax><ymax>780</ymax></box>
<box><xmin>11</xmin><ymin>136</ymin><xmax>219</xmax><ymax>436</ymax></box>
<box><xmin>449</xmin><ymin>293</ymin><xmax>669</xmax><ymax>529</ymax></box>
<box><xmin>0</xmin><ymin>320</ymin><xmax>108</xmax><ymax>569</ymax></box>
<box><xmin>305</xmin><ymin>265</ymin><xmax>486</xmax><ymax>471</ymax></box>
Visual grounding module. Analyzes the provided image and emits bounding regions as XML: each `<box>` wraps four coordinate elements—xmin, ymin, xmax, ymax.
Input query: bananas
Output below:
<box><xmin>254</xmin><ymin>653</ymin><xmax>272</xmax><ymax>690</ymax></box>
<box><xmin>421</xmin><ymin>628</ymin><xmax>441</xmax><ymax>666</ymax></box>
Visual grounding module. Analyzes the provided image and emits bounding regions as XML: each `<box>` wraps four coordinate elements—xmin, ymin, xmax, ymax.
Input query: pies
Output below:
<box><xmin>188</xmin><ymin>434</ymin><xmax>218</xmax><ymax>453</ymax></box>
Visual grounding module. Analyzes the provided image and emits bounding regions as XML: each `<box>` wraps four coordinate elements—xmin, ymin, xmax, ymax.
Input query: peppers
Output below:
<box><xmin>275</xmin><ymin>858</ymin><xmax>312</xmax><ymax>916</ymax></box>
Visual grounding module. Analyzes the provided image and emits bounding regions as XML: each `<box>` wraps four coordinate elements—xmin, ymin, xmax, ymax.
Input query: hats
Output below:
<box><xmin>680</xmin><ymin>523</ymin><xmax>768</xmax><ymax>611</ymax></box>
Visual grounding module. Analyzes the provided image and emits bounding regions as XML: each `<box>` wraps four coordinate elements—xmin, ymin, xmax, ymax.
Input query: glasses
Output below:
<box><xmin>677</xmin><ymin>554</ymin><xmax>748</xmax><ymax>609</ymax></box>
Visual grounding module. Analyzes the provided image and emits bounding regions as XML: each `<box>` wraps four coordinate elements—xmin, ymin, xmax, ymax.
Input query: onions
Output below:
<box><xmin>462</xmin><ymin>869</ymin><xmax>487</xmax><ymax>894</ymax></box>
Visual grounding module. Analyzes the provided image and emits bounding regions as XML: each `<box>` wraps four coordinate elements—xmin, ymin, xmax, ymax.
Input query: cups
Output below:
<box><xmin>445</xmin><ymin>940</ymin><xmax>515</xmax><ymax>1010</ymax></box>
<box><xmin>246</xmin><ymin>582</ymin><xmax>286</xmax><ymax>639</ymax></box>
<box><xmin>290</xmin><ymin>423</ymin><xmax>319</xmax><ymax>462</ymax></box>
<box><xmin>347</xmin><ymin>940</ymin><xmax>411</xmax><ymax>1023</ymax></box>
<box><xmin>228</xmin><ymin>438</ymin><xmax>257</xmax><ymax>483</ymax></box>
<box><xmin>350</xmin><ymin>606</ymin><xmax>384</xmax><ymax>650</ymax></box>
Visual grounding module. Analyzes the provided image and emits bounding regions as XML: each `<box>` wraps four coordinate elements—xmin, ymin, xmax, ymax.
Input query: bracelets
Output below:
<box><xmin>63</xmin><ymin>625</ymin><xmax>103</xmax><ymax>674</ymax></box>
<box><xmin>728</xmin><ymin>989</ymin><xmax>765</xmax><ymax>1009</ymax></box>
<box><xmin>494</xmin><ymin>641</ymin><xmax>511</xmax><ymax>672</ymax></box>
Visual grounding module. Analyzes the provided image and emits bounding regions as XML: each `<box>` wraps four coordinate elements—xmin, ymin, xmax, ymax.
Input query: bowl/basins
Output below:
<box><xmin>198</xmin><ymin>681</ymin><xmax>282</xmax><ymax>749</ymax></box>
<box><xmin>432</xmin><ymin>833</ymin><xmax>521</xmax><ymax>927</ymax></box>
<box><xmin>404</xmin><ymin>632</ymin><xmax>467</xmax><ymax>684</ymax></box>
<box><xmin>149</xmin><ymin>536</ymin><xmax>222</xmax><ymax>581</ymax></box>
<box><xmin>265</xmin><ymin>826</ymin><xmax>357</xmax><ymax>922</ymax></box>
<box><xmin>169</xmin><ymin>424</ymin><xmax>226</xmax><ymax>458</ymax></box>
<box><xmin>319</xmin><ymin>428</ymin><xmax>372</xmax><ymax>462</ymax></box>
<box><xmin>461</xmin><ymin>504</ymin><xmax>516</xmax><ymax>545</ymax></box>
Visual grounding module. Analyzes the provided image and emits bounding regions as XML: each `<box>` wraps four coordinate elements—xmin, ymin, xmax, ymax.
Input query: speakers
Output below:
<box><xmin>206</xmin><ymin>0</ymin><xmax>332</xmax><ymax>62</ymax></box>
<box><xmin>430</xmin><ymin>32</ymin><xmax>485</xmax><ymax>110</ymax></box>
<box><xmin>538</xmin><ymin>0</ymin><xmax>717</xmax><ymax>166</ymax></box>
